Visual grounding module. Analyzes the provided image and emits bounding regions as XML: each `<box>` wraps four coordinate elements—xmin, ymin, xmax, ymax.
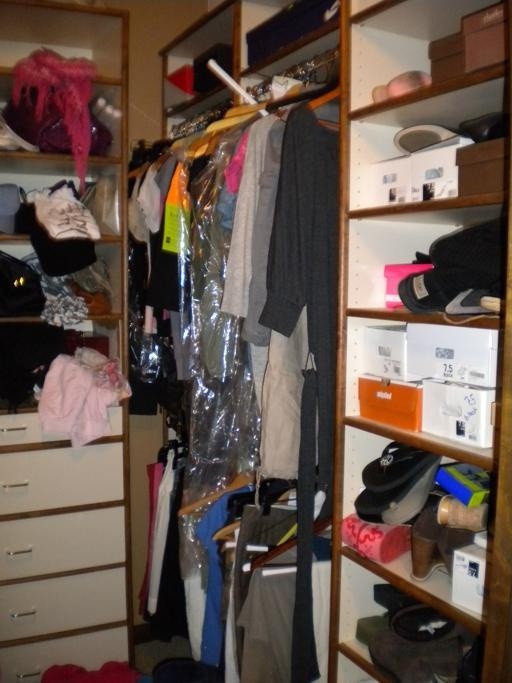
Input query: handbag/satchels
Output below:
<box><xmin>354</xmin><ymin>439</ymin><xmax>443</xmax><ymax>529</ymax></box>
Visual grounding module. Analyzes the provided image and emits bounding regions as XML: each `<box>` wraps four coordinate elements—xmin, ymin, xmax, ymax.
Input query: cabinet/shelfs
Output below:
<box><xmin>0</xmin><ymin>1</ymin><xmax>512</xmax><ymax>683</ymax></box>
